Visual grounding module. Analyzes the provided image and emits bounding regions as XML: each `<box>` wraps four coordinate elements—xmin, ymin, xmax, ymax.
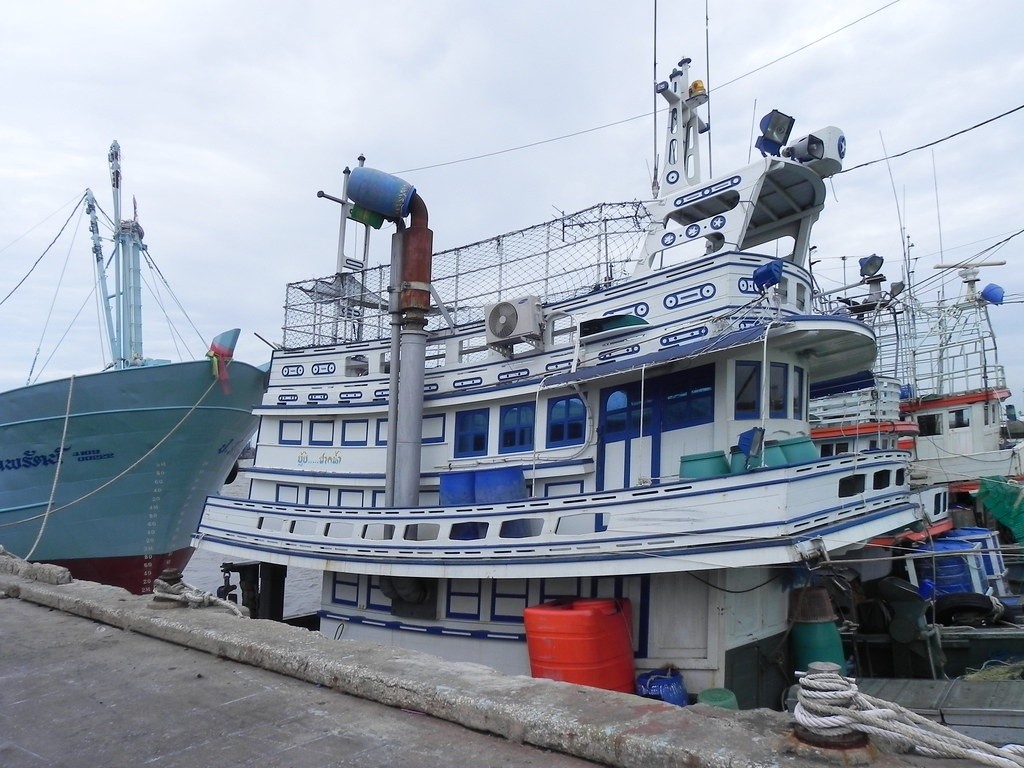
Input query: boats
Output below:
<box><xmin>154</xmin><ymin>0</ymin><xmax>1024</xmax><ymax>715</ymax></box>
<box><xmin>0</xmin><ymin>139</ymin><xmax>274</xmax><ymax>594</ymax></box>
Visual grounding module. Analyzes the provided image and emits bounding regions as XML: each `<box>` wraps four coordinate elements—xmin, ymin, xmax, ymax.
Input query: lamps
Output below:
<box><xmin>889</xmin><ymin>281</ymin><xmax>906</xmax><ymax>298</ymax></box>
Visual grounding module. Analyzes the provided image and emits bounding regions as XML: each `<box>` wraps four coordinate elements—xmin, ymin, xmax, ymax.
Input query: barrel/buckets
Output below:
<box><xmin>437</xmin><ymin>471</ymin><xmax>477</xmax><ymax>540</ymax></box>
<box><xmin>792</xmin><ymin>623</ymin><xmax>849</xmax><ymax>677</ymax></box>
<box><xmin>730</xmin><ymin>439</ymin><xmax>788</xmax><ymax>473</ymax></box>
<box><xmin>918</xmin><ymin>415</ymin><xmax>936</xmax><ymax>436</ymax></box>
<box><xmin>778</xmin><ymin>436</ymin><xmax>819</xmax><ymax>464</ymax></box>
<box><xmin>346</xmin><ymin>167</ymin><xmax>417</xmax><ymax>217</ymax></box>
<box><xmin>475</xmin><ymin>465</ymin><xmax>532</xmax><ymax>538</ymax></box>
<box><xmin>697</xmin><ymin>688</ymin><xmax>740</xmax><ymax>711</ymax></box>
<box><xmin>788</xmin><ymin>586</ymin><xmax>839</xmax><ymax>623</ymax></box>
<box><xmin>352</xmin><ymin>204</ymin><xmax>384</xmax><ymax>230</ymax></box>
<box><xmin>679</xmin><ymin>450</ymin><xmax>731</xmax><ymax>481</ymax></box>
<box><xmin>523</xmin><ymin>598</ymin><xmax>635</xmax><ymax>696</ymax></box>
<box><xmin>635</xmin><ymin>667</ymin><xmax>689</xmax><ymax>708</ymax></box>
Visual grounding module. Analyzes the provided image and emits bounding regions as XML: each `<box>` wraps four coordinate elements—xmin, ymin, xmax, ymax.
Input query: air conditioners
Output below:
<box><xmin>484</xmin><ymin>295</ymin><xmax>542</xmax><ymax>345</ymax></box>
